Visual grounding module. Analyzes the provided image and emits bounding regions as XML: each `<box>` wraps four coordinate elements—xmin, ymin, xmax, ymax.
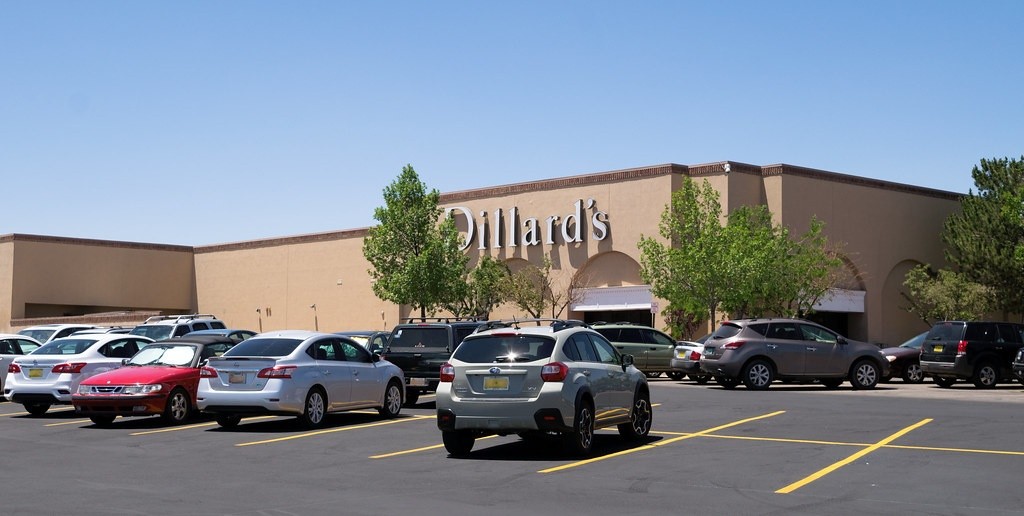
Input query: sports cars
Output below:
<box><xmin>69</xmin><ymin>334</ymin><xmax>244</xmax><ymax>426</ymax></box>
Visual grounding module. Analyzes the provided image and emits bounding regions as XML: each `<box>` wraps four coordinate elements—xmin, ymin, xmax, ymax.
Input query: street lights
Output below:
<box><xmin>309</xmin><ymin>305</ymin><xmax>320</xmax><ymax>331</ymax></box>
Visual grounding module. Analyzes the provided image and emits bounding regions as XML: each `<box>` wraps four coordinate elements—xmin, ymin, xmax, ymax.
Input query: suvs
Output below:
<box><xmin>918</xmin><ymin>320</ymin><xmax>1024</xmax><ymax>390</ymax></box>
<box><xmin>701</xmin><ymin>315</ymin><xmax>893</xmax><ymax>391</ymax></box>
<box><xmin>586</xmin><ymin>321</ymin><xmax>688</xmax><ymax>381</ymax></box>
<box><xmin>125</xmin><ymin>314</ymin><xmax>227</xmax><ymax>342</ymax></box>
<box><xmin>382</xmin><ymin>316</ymin><xmax>517</xmax><ymax>407</ymax></box>
<box><xmin>434</xmin><ymin>317</ymin><xmax>654</xmax><ymax>460</ymax></box>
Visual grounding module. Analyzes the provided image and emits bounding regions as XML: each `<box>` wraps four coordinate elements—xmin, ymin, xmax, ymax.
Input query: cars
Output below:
<box><xmin>314</xmin><ymin>330</ymin><xmax>396</xmax><ymax>360</ymax></box>
<box><xmin>195</xmin><ymin>328</ymin><xmax>407</xmax><ymax>426</ymax></box>
<box><xmin>0</xmin><ymin>332</ymin><xmax>44</xmax><ymax>402</ymax></box>
<box><xmin>883</xmin><ymin>331</ymin><xmax>937</xmax><ymax>384</ymax></box>
<box><xmin>181</xmin><ymin>328</ymin><xmax>259</xmax><ymax>358</ymax></box>
<box><xmin>669</xmin><ymin>332</ymin><xmax>714</xmax><ymax>386</ymax></box>
<box><xmin>55</xmin><ymin>325</ymin><xmax>146</xmax><ymax>359</ymax></box>
<box><xmin>1012</xmin><ymin>348</ymin><xmax>1024</xmax><ymax>388</ymax></box>
<box><xmin>11</xmin><ymin>323</ymin><xmax>105</xmax><ymax>356</ymax></box>
<box><xmin>2</xmin><ymin>334</ymin><xmax>159</xmax><ymax>417</ymax></box>
